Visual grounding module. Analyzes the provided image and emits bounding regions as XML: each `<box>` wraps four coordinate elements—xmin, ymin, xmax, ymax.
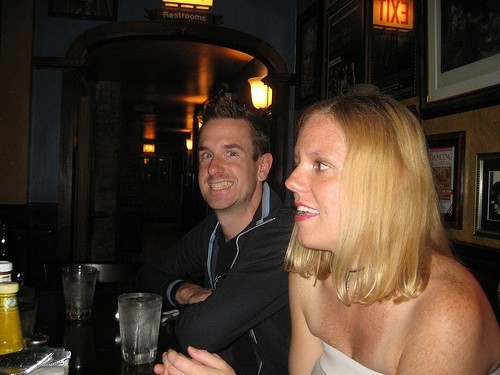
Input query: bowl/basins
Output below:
<box><xmin>0</xmin><ymin>347</ymin><xmax>72</xmax><ymax>375</ymax></box>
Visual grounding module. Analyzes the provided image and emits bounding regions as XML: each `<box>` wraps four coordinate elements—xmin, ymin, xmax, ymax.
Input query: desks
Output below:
<box><xmin>0</xmin><ymin>283</ymin><xmax>188</xmax><ymax>375</ymax></box>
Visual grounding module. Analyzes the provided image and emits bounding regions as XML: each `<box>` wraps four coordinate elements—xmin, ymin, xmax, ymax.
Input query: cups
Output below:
<box><xmin>62</xmin><ymin>265</ymin><xmax>99</xmax><ymax>321</ymax></box>
<box><xmin>16</xmin><ymin>296</ymin><xmax>37</xmax><ymax>338</ymax></box>
<box><xmin>117</xmin><ymin>292</ymin><xmax>163</xmax><ymax>363</ymax></box>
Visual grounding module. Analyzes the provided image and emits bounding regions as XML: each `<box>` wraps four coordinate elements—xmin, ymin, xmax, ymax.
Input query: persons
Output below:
<box><xmin>136</xmin><ymin>93</ymin><xmax>297</xmax><ymax>374</ymax></box>
<box><xmin>153</xmin><ymin>86</ymin><xmax>500</xmax><ymax>375</ymax></box>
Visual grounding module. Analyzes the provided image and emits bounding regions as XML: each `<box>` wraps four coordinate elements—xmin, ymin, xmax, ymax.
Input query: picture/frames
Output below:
<box><xmin>295</xmin><ymin>0</ymin><xmax>324</xmax><ymax>111</ymax></box>
<box><xmin>472</xmin><ymin>152</ymin><xmax>500</xmax><ymax>240</ymax></box>
<box><xmin>419</xmin><ymin>0</ymin><xmax>500</xmax><ymax>120</ymax></box>
<box><xmin>324</xmin><ymin>0</ymin><xmax>366</xmax><ymax>100</ymax></box>
<box><xmin>424</xmin><ymin>130</ymin><xmax>466</xmax><ymax>230</ymax></box>
<box><xmin>365</xmin><ymin>0</ymin><xmax>417</xmax><ymax>106</ymax></box>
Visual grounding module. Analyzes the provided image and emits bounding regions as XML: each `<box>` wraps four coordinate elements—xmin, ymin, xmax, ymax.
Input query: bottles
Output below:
<box><xmin>0</xmin><ymin>261</ymin><xmax>12</xmax><ymax>281</ymax></box>
<box><xmin>0</xmin><ymin>281</ymin><xmax>25</xmax><ymax>355</ymax></box>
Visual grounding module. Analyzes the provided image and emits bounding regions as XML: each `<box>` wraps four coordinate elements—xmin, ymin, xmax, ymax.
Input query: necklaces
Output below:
<box><xmin>346</xmin><ymin>269</ymin><xmax>363</xmax><ymax>289</ymax></box>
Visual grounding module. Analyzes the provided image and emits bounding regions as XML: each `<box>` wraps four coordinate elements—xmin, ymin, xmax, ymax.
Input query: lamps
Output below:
<box><xmin>247</xmin><ymin>76</ymin><xmax>273</xmax><ymax>110</ymax></box>
<box><xmin>142</xmin><ymin>143</ymin><xmax>156</xmax><ymax>153</ymax></box>
<box><xmin>186</xmin><ymin>138</ymin><xmax>193</xmax><ymax>151</ymax></box>
